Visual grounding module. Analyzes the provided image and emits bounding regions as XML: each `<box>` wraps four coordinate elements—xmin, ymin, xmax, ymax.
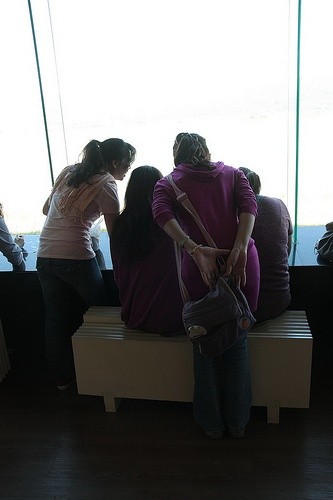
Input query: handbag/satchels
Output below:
<box><xmin>181</xmin><ymin>275</ymin><xmax>256</xmax><ymax>354</ymax></box>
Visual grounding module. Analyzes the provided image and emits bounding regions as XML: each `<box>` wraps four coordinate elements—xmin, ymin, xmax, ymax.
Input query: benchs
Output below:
<box><xmin>70</xmin><ymin>305</ymin><xmax>314</xmax><ymax>424</ymax></box>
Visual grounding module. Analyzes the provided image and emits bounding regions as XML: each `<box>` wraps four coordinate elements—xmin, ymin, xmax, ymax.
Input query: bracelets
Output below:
<box><xmin>189</xmin><ymin>244</ymin><xmax>202</xmax><ymax>256</ymax></box>
<box><xmin>176</xmin><ymin>235</ymin><xmax>190</xmax><ymax>248</ymax></box>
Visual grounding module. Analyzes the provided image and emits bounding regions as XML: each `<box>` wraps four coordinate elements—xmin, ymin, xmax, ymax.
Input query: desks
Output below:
<box><xmin>0</xmin><ymin>248</ymin><xmax>333</xmax><ymax>370</ymax></box>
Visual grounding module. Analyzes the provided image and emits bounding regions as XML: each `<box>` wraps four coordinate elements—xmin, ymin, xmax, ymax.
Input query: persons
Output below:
<box><xmin>37</xmin><ymin>138</ymin><xmax>136</xmax><ymax>389</ymax></box>
<box><xmin>109</xmin><ymin>167</ymin><xmax>185</xmax><ymax>336</ymax></box>
<box><xmin>0</xmin><ymin>203</ymin><xmax>25</xmax><ymax>383</ymax></box>
<box><xmin>152</xmin><ymin>133</ymin><xmax>260</xmax><ymax>438</ymax></box>
<box><xmin>236</xmin><ymin>166</ymin><xmax>292</xmax><ymax>328</ymax></box>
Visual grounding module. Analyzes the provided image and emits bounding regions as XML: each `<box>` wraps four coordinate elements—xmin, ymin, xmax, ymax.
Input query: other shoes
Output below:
<box><xmin>206</xmin><ymin>428</ymin><xmax>224</xmax><ymax>439</ymax></box>
<box><xmin>229</xmin><ymin>429</ymin><xmax>244</xmax><ymax>438</ymax></box>
